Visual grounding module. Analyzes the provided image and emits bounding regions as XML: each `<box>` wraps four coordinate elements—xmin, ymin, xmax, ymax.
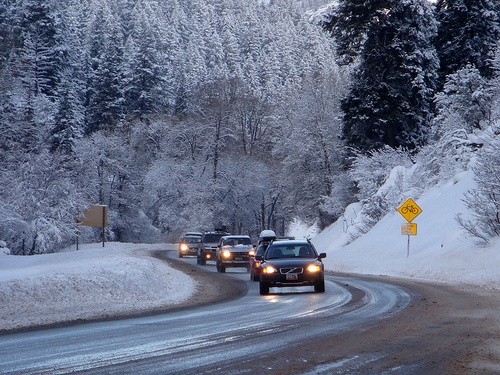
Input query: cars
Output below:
<box><xmin>249</xmin><ymin>236</ymin><xmax>295</xmax><ymax>279</ymax></box>
<box><xmin>183</xmin><ymin>232</ymin><xmax>202</xmax><ymax>236</ymax></box>
<box><xmin>178</xmin><ymin>236</ymin><xmax>200</xmax><ymax>258</ymax></box>
<box><xmin>216</xmin><ymin>235</ymin><xmax>254</xmax><ymax>271</ymax></box>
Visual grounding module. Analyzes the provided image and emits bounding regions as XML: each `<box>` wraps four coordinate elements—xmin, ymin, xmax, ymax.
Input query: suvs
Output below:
<box><xmin>255</xmin><ymin>237</ymin><xmax>326</xmax><ymax>295</ymax></box>
<box><xmin>197</xmin><ymin>232</ymin><xmax>230</xmax><ymax>265</ymax></box>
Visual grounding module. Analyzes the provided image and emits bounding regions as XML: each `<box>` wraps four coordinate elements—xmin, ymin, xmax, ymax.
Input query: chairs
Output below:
<box><xmin>269</xmin><ymin>249</ymin><xmax>282</xmax><ymax>258</ymax></box>
